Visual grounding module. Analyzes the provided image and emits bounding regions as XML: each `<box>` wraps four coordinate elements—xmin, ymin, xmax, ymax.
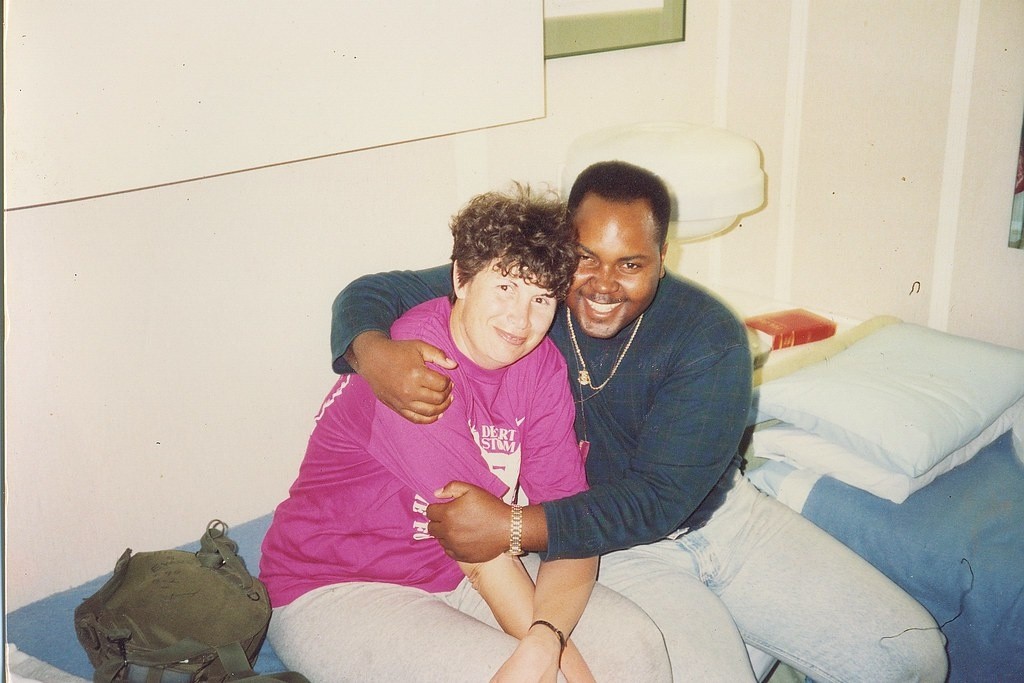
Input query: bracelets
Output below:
<box><xmin>507</xmin><ymin>503</ymin><xmax>526</xmax><ymax>560</ymax></box>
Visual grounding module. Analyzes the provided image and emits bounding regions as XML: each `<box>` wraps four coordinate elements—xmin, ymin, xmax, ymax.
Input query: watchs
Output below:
<box><xmin>528</xmin><ymin>620</ymin><xmax>565</xmax><ymax>651</ymax></box>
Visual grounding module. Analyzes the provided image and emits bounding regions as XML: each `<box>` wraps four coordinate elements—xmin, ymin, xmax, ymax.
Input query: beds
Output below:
<box><xmin>0</xmin><ymin>313</ymin><xmax>1024</xmax><ymax>683</ymax></box>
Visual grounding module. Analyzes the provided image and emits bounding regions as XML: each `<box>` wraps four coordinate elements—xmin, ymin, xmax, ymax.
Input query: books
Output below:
<box><xmin>743</xmin><ymin>307</ymin><xmax>837</xmax><ymax>351</ymax></box>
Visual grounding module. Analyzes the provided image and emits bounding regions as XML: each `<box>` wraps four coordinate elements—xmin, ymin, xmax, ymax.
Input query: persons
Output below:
<box><xmin>330</xmin><ymin>162</ymin><xmax>949</xmax><ymax>683</ymax></box>
<box><xmin>258</xmin><ymin>181</ymin><xmax>673</xmax><ymax>683</ymax></box>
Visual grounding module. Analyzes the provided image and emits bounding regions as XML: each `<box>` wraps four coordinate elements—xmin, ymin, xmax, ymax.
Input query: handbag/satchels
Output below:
<box><xmin>74</xmin><ymin>519</ymin><xmax>308</xmax><ymax>683</ymax></box>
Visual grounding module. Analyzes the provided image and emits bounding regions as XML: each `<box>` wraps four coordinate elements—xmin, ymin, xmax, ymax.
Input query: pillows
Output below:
<box><xmin>751</xmin><ymin>322</ymin><xmax>1024</xmax><ymax>478</ymax></box>
<box><xmin>753</xmin><ymin>397</ymin><xmax>1024</xmax><ymax>505</ymax></box>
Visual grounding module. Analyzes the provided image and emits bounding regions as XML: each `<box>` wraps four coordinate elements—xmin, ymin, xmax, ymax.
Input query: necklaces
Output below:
<box><xmin>565</xmin><ymin>305</ymin><xmax>645</xmax><ymax>442</ymax></box>
